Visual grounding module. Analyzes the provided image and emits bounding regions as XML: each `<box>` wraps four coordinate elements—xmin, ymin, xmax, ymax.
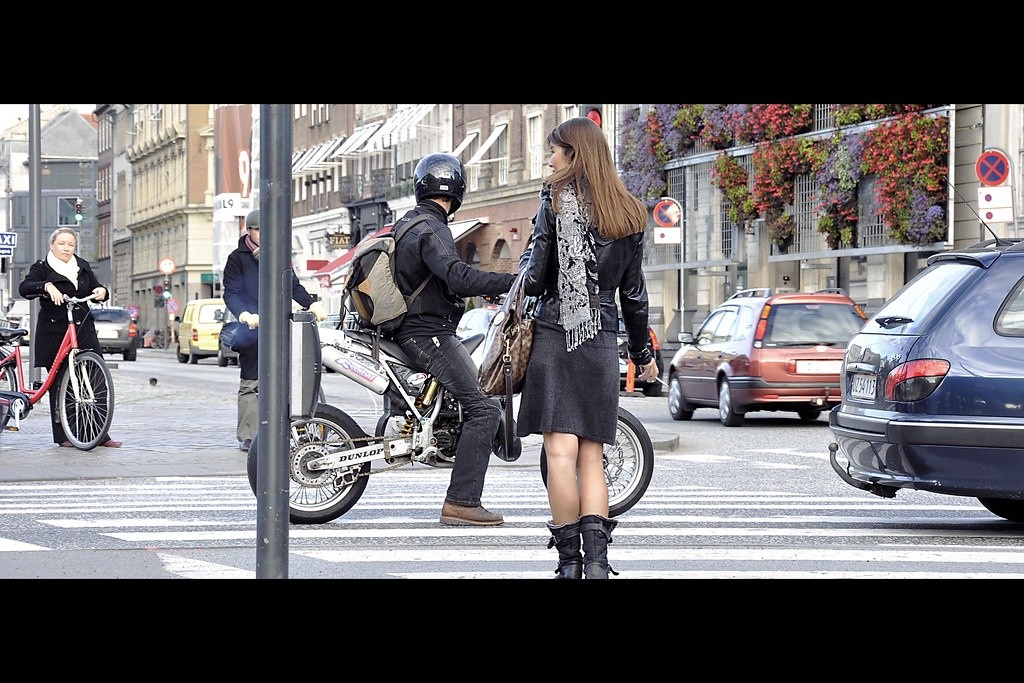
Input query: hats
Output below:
<box><xmin>245</xmin><ymin>209</ymin><xmax>263</xmax><ymax>228</ymax></box>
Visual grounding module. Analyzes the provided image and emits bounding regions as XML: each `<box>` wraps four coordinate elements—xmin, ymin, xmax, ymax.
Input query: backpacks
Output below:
<box><xmin>332</xmin><ymin>214</ymin><xmax>450</xmax><ymax>337</ymax></box>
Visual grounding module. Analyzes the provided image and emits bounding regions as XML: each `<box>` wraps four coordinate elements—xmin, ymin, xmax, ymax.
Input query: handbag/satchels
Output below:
<box><xmin>473</xmin><ymin>267</ymin><xmax>537</xmax><ymax>398</ymax></box>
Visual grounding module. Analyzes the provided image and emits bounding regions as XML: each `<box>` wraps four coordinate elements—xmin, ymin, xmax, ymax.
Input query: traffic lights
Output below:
<box><xmin>81</xmin><ymin>206</ymin><xmax>87</xmax><ymax>221</ymax></box>
<box><xmin>75</xmin><ymin>203</ymin><xmax>82</xmax><ymax>222</ymax></box>
<box><xmin>580</xmin><ymin>104</ymin><xmax>604</xmax><ymax>129</ymax></box>
<box><xmin>162</xmin><ymin>280</ymin><xmax>170</xmax><ymax>300</ymax></box>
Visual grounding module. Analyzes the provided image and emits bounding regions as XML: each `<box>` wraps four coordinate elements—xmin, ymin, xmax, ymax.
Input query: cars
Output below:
<box><xmin>668</xmin><ymin>285</ymin><xmax>871</xmax><ymax>428</ymax></box>
<box><xmin>213</xmin><ymin>304</ymin><xmax>242</xmax><ymax>369</ymax></box>
<box><xmin>18</xmin><ymin>313</ymin><xmax>32</xmax><ymax>346</ymax></box>
<box><xmin>616</xmin><ymin>307</ymin><xmax>665</xmax><ymax>398</ymax></box>
<box><xmin>828</xmin><ymin>234</ymin><xmax>1024</xmax><ymax>523</ymax></box>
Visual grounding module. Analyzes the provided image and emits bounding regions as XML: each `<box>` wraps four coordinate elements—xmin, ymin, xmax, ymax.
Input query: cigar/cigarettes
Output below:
<box><xmin>655</xmin><ymin>377</ymin><xmax>669</xmax><ymax>387</ymax></box>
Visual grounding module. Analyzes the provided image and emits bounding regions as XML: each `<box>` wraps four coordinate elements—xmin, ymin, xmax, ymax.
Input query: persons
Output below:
<box><xmin>514</xmin><ymin>116</ymin><xmax>659</xmax><ymax>580</ymax></box>
<box><xmin>389</xmin><ymin>152</ymin><xmax>519</xmax><ymax>526</ymax></box>
<box><xmin>222</xmin><ymin>210</ymin><xmax>328</xmax><ymax>453</ymax></box>
<box><xmin>17</xmin><ymin>226</ymin><xmax>123</xmax><ymax>448</ymax></box>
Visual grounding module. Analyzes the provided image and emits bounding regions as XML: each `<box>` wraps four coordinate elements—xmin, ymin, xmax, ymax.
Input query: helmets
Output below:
<box><xmin>414</xmin><ymin>152</ymin><xmax>468</xmax><ymax>209</ymax></box>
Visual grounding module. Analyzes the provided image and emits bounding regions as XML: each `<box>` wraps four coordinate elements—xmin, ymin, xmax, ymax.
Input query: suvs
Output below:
<box><xmin>90</xmin><ymin>304</ymin><xmax>139</xmax><ymax>363</ymax></box>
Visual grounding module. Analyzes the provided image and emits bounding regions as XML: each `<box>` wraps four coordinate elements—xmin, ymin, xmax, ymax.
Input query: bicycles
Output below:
<box><xmin>0</xmin><ymin>288</ymin><xmax>116</xmax><ymax>452</ymax></box>
<box><xmin>149</xmin><ymin>329</ymin><xmax>172</xmax><ymax>349</ymax></box>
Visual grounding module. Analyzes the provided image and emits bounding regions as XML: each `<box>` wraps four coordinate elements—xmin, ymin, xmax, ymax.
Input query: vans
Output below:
<box><xmin>172</xmin><ymin>298</ymin><xmax>227</xmax><ymax>367</ymax></box>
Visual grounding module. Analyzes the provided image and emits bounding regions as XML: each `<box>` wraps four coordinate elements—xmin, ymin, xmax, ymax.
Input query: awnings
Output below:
<box><xmin>448</xmin><ymin>220</ymin><xmax>483</xmax><ymax>242</ymax></box>
<box><xmin>310</xmin><ymin>226</ymin><xmax>392</xmax><ymax>289</ymax></box>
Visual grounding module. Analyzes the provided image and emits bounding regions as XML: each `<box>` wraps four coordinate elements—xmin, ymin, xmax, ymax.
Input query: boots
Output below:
<box><xmin>581</xmin><ymin>513</ymin><xmax>619</xmax><ymax>578</ymax></box>
<box><xmin>545</xmin><ymin>520</ymin><xmax>584</xmax><ymax>580</ymax></box>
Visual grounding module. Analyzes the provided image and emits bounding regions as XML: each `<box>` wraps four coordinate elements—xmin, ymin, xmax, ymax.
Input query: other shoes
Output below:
<box><xmin>439</xmin><ymin>500</ymin><xmax>504</xmax><ymax>529</ymax></box>
<box><xmin>98</xmin><ymin>439</ymin><xmax>124</xmax><ymax>448</ymax></box>
<box><xmin>59</xmin><ymin>440</ymin><xmax>75</xmax><ymax>447</ymax></box>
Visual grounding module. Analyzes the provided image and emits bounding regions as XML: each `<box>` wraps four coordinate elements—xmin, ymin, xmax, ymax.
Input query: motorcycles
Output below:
<box><xmin>246</xmin><ymin>292</ymin><xmax>657</xmax><ymax>526</ymax></box>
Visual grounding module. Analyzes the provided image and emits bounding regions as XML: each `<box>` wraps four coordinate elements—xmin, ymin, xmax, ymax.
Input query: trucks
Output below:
<box><xmin>5</xmin><ymin>299</ymin><xmax>30</xmax><ymax>327</ymax></box>
